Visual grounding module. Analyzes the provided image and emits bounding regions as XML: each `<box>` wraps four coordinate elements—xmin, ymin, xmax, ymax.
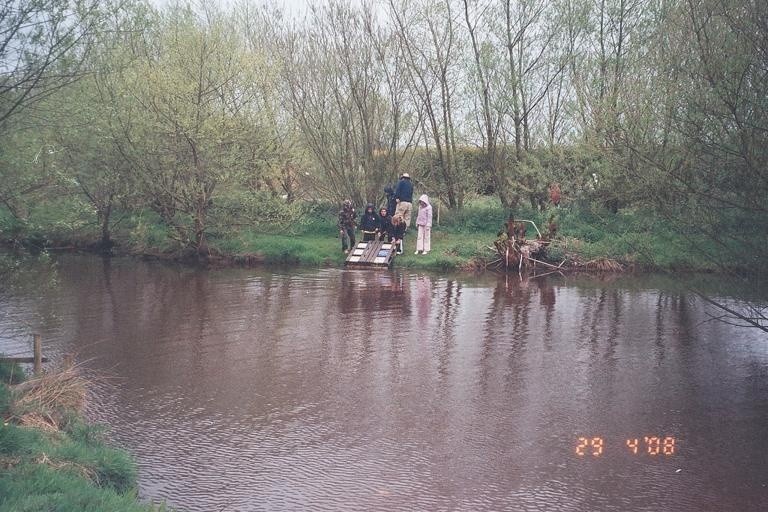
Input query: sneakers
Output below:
<box><xmin>414</xmin><ymin>250</ymin><xmax>429</xmax><ymax>255</ymax></box>
<box><xmin>396</xmin><ymin>251</ymin><xmax>400</xmax><ymax>254</ymax></box>
<box><xmin>343</xmin><ymin>249</ymin><xmax>349</xmax><ymax>255</ymax></box>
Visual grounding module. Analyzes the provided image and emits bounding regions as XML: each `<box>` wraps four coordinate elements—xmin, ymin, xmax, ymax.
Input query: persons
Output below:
<box><xmin>383</xmin><ymin>186</ymin><xmax>398</xmax><ymax>216</ymax></box>
<box><xmin>414</xmin><ymin>194</ymin><xmax>434</xmax><ymax>255</ymax></box>
<box><xmin>390</xmin><ymin>213</ymin><xmax>407</xmax><ymax>255</ymax></box>
<box><xmin>338</xmin><ymin>270</ymin><xmax>433</xmax><ymax>333</ymax></box>
<box><xmin>359</xmin><ymin>203</ymin><xmax>380</xmax><ymax>242</ymax></box>
<box><xmin>378</xmin><ymin>207</ymin><xmax>393</xmax><ymax>241</ymax></box>
<box><xmin>337</xmin><ymin>198</ymin><xmax>359</xmax><ymax>255</ymax></box>
<box><xmin>394</xmin><ymin>172</ymin><xmax>413</xmax><ymax>231</ymax></box>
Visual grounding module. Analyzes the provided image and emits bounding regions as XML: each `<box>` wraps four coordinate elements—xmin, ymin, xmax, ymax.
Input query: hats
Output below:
<box><xmin>399</xmin><ymin>173</ymin><xmax>411</xmax><ymax>180</ymax></box>
